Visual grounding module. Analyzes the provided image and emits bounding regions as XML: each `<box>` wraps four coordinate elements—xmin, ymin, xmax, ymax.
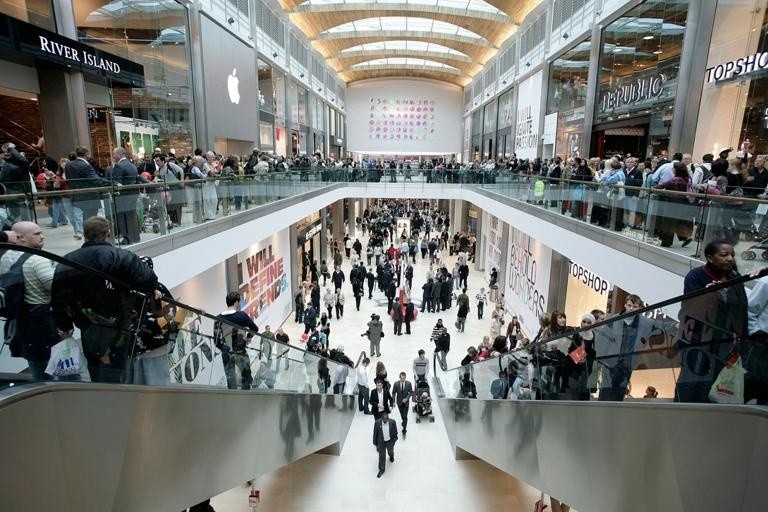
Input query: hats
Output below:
<box><xmin>719</xmin><ymin>147</ymin><xmax>731</xmax><ymax>155</ymax></box>
<box><xmin>580</xmin><ymin>313</ymin><xmax>595</xmax><ymax>329</ymax></box>
<box><xmin>703</xmin><ymin>154</ymin><xmax>713</xmax><ymax>161</ymax></box>
<box><xmin>253</xmin><ymin>148</ymin><xmax>260</xmax><ymax>152</ymax></box>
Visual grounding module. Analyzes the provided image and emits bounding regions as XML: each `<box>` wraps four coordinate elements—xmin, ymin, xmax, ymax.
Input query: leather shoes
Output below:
<box><xmin>377</xmin><ymin>471</ymin><xmax>383</xmax><ymax>477</ymax></box>
<box><xmin>45</xmin><ymin>223</ymin><xmax>68</xmax><ymax>228</ymax></box>
<box><xmin>390</xmin><ymin>456</ymin><xmax>394</xmax><ymax>462</ymax></box>
<box><xmin>368</xmin><ymin>411</ymin><xmax>372</xmax><ymax>415</ymax></box>
<box><xmin>116</xmin><ymin>239</ymin><xmax>128</xmax><ymax>245</ymax></box>
<box><xmin>153</xmin><ymin>224</ymin><xmax>159</xmax><ymax>233</ymax></box>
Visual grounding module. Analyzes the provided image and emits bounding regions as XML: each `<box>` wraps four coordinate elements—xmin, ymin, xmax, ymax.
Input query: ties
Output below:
<box><xmin>402</xmin><ymin>383</ymin><xmax>403</xmax><ymax>392</ymax></box>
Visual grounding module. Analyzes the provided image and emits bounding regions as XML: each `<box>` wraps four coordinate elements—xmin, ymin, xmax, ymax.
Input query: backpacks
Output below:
<box><xmin>698</xmin><ymin>165</ymin><xmax>713</xmax><ymax>184</ymax></box>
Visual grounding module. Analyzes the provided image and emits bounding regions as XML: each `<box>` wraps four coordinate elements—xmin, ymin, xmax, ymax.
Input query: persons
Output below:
<box><xmin>372</xmin><ymin>414</ymin><xmax>398</xmax><ymax>478</ymax></box>
<box><xmin>189</xmin><ymin>499</ymin><xmax>216</xmax><ymax>512</ymax></box>
<box><xmin>0</xmin><ymin>134</ymin><xmax>768</xmax><ymax>434</ymax></box>
<box><xmin>549</xmin><ymin>496</ymin><xmax>570</xmax><ymax>512</ymax></box>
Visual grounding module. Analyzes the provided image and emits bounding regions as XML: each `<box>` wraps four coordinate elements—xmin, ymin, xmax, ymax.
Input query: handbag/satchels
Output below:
<box><xmin>607</xmin><ymin>181</ymin><xmax>626</xmax><ymax>200</ymax></box>
<box><xmin>570</xmin><ymin>345</ymin><xmax>587</xmax><ymax>364</ymax></box>
<box><xmin>0</xmin><ymin>263</ymin><xmax>24</xmax><ymax>320</ymax></box>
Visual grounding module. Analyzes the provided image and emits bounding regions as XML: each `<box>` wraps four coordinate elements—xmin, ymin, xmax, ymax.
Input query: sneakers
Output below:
<box><xmin>682</xmin><ymin>239</ymin><xmax>692</xmax><ymax>247</ymax></box>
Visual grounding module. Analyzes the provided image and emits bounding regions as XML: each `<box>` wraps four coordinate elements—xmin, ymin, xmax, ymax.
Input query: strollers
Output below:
<box><xmin>303</xmin><ymin>329</ymin><xmax>321</xmax><ymax>356</ymax></box>
<box><xmin>732</xmin><ymin>195</ymin><xmax>760</xmax><ymax>240</ymax></box>
<box><xmin>139</xmin><ymin>181</ymin><xmax>174</xmax><ymax>234</ymax></box>
<box><xmin>527</xmin><ymin>177</ymin><xmax>548</xmax><ymax>204</ymax></box>
<box><xmin>741</xmin><ymin>235</ymin><xmax>767</xmax><ymax>262</ymax></box>
<box><xmin>412</xmin><ymin>379</ymin><xmax>435</xmax><ymax>423</ymax></box>
